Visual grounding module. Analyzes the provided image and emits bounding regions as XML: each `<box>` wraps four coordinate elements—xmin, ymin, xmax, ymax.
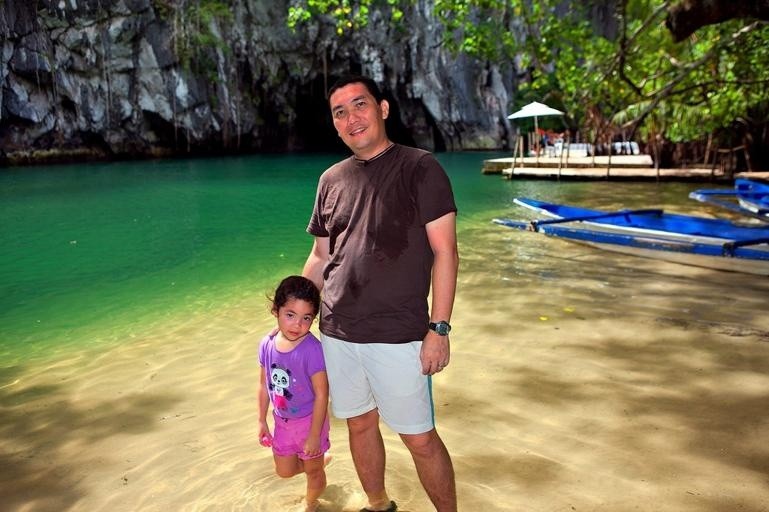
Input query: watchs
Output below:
<box><xmin>427</xmin><ymin>320</ymin><xmax>451</xmax><ymax>336</ymax></box>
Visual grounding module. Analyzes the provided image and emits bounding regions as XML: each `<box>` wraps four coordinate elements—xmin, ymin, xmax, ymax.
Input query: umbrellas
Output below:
<box><xmin>504</xmin><ymin>99</ymin><xmax>567</xmax><ymax>158</ymax></box>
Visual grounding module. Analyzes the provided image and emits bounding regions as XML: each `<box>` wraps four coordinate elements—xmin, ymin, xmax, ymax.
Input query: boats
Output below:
<box><xmin>493</xmin><ymin>177</ymin><xmax>769</xmax><ymax>260</ymax></box>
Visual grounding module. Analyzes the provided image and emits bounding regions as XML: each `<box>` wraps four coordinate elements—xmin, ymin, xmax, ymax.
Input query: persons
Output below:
<box><xmin>256</xmin><ymin>274</ymin><xmax>329</xmax><ymax>512</ymax></box>
<box><xmin>530</xmin><ymin>125</ymin><xmax>571</xmax><ymax>155</ymax></box>
<box><xmin>266</xmin><ymin>75</ymin><xmax>462</xmax><ymax>512</ymax></box>
